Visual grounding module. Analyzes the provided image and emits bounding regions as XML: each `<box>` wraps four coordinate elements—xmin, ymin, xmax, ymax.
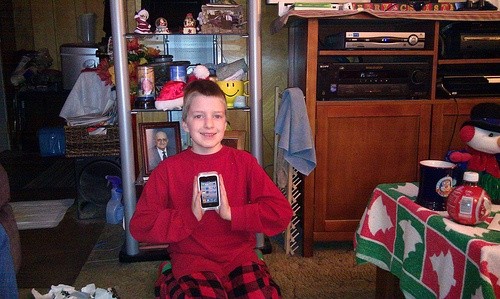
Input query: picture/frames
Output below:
<box><xmin>139</xmin><ymin>121</ymin><xmax>182</xmax><ymax>177</ymax></box>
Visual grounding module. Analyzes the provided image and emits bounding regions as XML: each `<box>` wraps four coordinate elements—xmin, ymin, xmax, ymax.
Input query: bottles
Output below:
<box><xmin>446</xmin><ymin>171</ymin><xmax>492</xmax><ymax>226</ymax></box>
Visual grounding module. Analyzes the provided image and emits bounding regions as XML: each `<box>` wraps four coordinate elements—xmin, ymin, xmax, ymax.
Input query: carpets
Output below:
<box><xmin>73</xmin><ymin>222</ymin><xmax>378</xmax><ymax>299</ymax></box>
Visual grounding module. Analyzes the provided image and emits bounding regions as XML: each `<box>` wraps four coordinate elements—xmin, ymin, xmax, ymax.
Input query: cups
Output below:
<box><xmin>419</xmin><ymin>160</ymin><xmax>459</xmax><ymax>211</ymax></box>
<box><xmin>216</xmin><ymin>80</ymin><xmax>250</xmax><ymax>108</ymax></box>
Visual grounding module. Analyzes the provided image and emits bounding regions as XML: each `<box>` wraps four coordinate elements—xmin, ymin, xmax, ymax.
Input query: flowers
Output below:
<box><xmin>100</xmin><ymin>40</ymin><xmax>157</xmax><ymax>94</ymax></box>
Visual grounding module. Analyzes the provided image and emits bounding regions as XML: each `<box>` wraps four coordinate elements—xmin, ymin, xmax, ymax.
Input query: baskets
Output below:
<box><xmin>63</xmin><ymin>122</ymin><xmax>120</xmax><ymax>160</ymax></box>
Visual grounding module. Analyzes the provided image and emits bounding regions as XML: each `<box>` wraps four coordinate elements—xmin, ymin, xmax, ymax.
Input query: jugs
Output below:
<box><xmin>76</xmin><ymin>13</ymin><xmax>97</xmax><ymax>44</ymax></box>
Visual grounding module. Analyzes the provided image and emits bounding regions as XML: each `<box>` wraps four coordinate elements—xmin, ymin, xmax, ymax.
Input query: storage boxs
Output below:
<box><xmin>64</xmin><ymin>126</ymin><xmax>121</xmax><ymax>157</ymax></box>
<box><xmin>37</xmin><ymin>127</ymin><xmax>64</xmax><ymax>157</ymax></box>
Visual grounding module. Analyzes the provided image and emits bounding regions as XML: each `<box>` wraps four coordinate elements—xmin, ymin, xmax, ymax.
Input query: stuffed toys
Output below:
<box><xmin>449</xmin><ymin>102</ymin><xmax>500</xmax><ymax>204</ymax></box>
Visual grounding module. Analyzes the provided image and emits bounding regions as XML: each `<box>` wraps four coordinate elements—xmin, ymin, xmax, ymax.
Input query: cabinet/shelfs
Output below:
<box><xmin>120</xmin><ymin>0</ymin><xmax>258</xmax><ymax>248</ymax></box>
<box><xmin>288</xmin><ymin>12</ymin><xmax>500</xmax><ymax>257</ymax></box>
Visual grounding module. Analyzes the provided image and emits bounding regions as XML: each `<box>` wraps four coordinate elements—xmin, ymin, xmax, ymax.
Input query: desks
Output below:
<box><xmin>354</xmin><ymin>181</ymin><xmax>500</xmax><ymax>299</ymax></box>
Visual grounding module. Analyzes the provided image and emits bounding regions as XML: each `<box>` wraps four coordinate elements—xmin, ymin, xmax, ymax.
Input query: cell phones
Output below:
<box><xmin>199</xmin><ymin>174</ymin><xmax>220</xmax><ymax>210</ymax></box>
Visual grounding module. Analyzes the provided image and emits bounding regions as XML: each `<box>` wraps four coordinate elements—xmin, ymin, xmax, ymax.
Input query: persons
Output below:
<box><xmin>142</xmin><ymin>78</ymin><xmax>154</xmax><ymax>95</ymax></box>
<box><xmin>128</xmin><ymin>78</ymin><xmax>294</xmax><ymax>299</ymax></box>
<box><xmin>0</xmin><ymin>163</ymin><xmax>23</xmax><ymax>299</ymax></box>
<box><xmin>147</xmin><ymin>131</ymin><xmax>177</xmax><ymax>170</ymax></box>
<box><xmin>134</xmin><ymin>8</ymin><xmax>152</xmax><ymax>34</ymax></box>
